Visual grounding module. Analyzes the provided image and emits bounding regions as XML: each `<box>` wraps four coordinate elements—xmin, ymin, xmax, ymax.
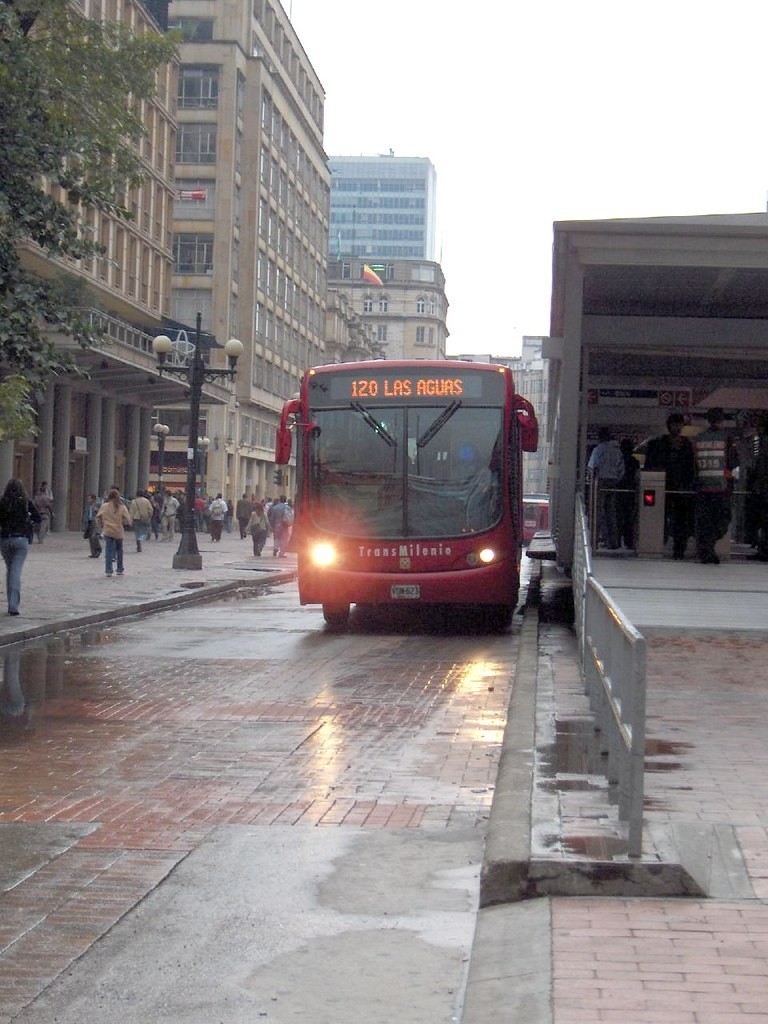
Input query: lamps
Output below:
<box><xmin>214</xmin><ymin>433</ymin><xmax>219</xmax><ymax>450</ymax></box>
<box><xmin>249</xmin><ymin>441</ymin><xmax>255</xmax><ymax>452</ymax></box>
<box><xmin>237</xmin><ymin>438</ymin><xmax>244</xmax><ymax>449</ymax></box>
<box><xmin>227</xmin><ymin>434</ymin><xmax>233</xmax><ymax>446</ymax></box>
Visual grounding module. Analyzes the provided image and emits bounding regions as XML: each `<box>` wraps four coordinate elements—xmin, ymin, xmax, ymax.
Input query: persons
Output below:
<box><xmin>0</xmin><ymin>479</ymin><xmax>42</xmax><ymax>615</ymax></box>
<box><xmin>586</xmin><ymin>407</ymin><xmax>768</xmax><ymax>565</ymax></box>
<box><xmin>83</xmin><ymin>486</ymin><xmax>234</xmax><ymax>558</ymax></box>
<box><xmin>33</xmin><ymin>481</ymin><xmax>55</xmax><ymax>544</ymax></box>
<box><xmin>236</xmin><ymin>493</ymin><xmax>294</xmax><ymax>558</ymax></box>
<box><xmin>95</xmin><ymin>489</ymin><xmax>130</xmax><ymax>577</ymax></box>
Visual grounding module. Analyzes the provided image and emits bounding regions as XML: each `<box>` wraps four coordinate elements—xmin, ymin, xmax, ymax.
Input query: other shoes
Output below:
<box><xmin>88</xmin><ymin>555</ymin><xmax>99</xmax><ymax>558</ymax></box>
<box><xmin>11</xmin><ymin>612</ymin><xmax>19</xmax><ymax>616</ymax></box>
<box><xmin>116</xmin><ymin>571</ymin><xmax>124</xmax><ymax>575</ymax></box>
<box><xmin>106</xmin><ymin>573</ymin><xmax>112</xmax><ymax>577</ymax></box>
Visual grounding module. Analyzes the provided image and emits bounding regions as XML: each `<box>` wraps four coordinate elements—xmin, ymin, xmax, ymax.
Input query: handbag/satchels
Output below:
<box><xmin>282</xmin><ymin>511</ymin><xmax>294</xmax><ymax>527</ymax></box>
<box><xmin>213</xmin><ymin>506</ymin><xmax>222</xmax><ymax>516</ymax></box>
<box><xmin>251</xmin><ymin>524</ymin><xmax>259</xmax><ymax>536</ymax></box>
<box><xmin>140</xmin><ymin>515</ymin><xmax>151</xmax><ymax>527</ymax></box>
<box><xmin>24</xmin><ymin>500</ymin><xmax>35</xmax><ymax>528</ymax></box>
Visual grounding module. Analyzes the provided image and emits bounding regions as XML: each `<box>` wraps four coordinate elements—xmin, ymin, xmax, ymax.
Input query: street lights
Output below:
<box><xmin>197</xmin><ymin>437</ymin><xmax>210</xmax><ymax>499</ymax></box>
<box><xmin>154</xmin><ymin>424</ymin><xmax>170</xmax><ymax>493</ymax></box>
<box><xmin>153</xmin><ymin>312</ymin><xmax>243</xmax><ymax>571</ymax></box>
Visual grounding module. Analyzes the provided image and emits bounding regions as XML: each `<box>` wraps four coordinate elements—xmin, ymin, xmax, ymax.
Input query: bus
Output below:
<box><xmin>275</xmin><ymin>360</ymin><xmax>539</xmax><ymax>626</ymax></box>
<box><xmin>523</xmin><ymin>493</ymin><xmax>549</xmax><ymax>545</ymax></box>
